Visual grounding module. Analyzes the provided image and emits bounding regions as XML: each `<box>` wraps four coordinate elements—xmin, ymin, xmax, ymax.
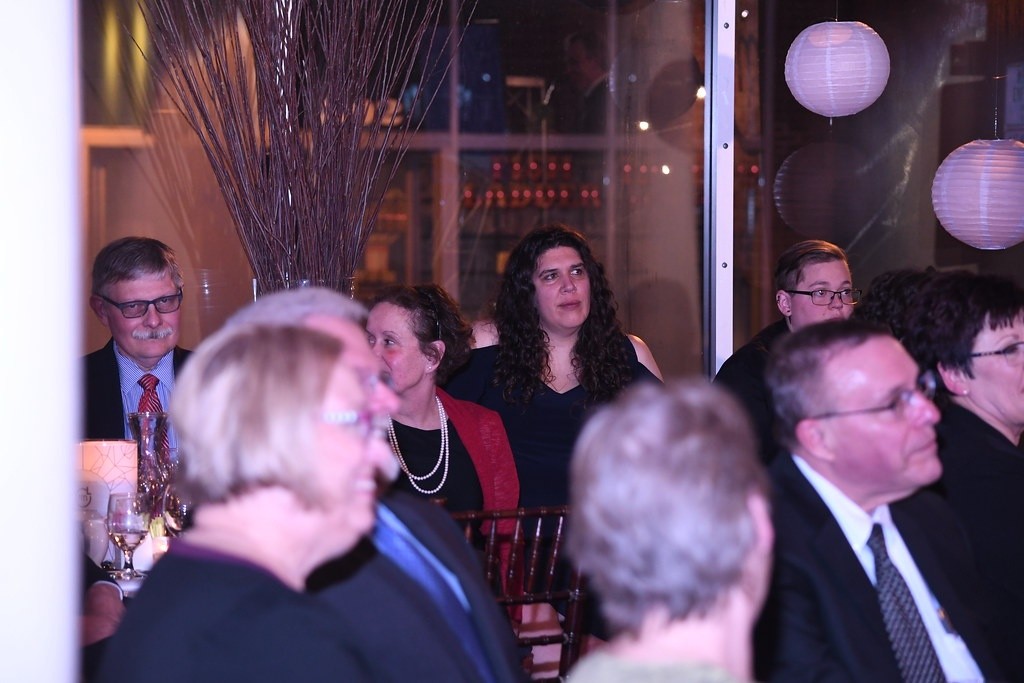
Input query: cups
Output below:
<box><xmin>163</xmin><ymin>483</ymin><xmax>195</xmax><ymax>540</ymax></box>
<box><xmin>159</xmin><ymin>446</ymin><xmax>180</xmax><ymax>484</ymax></box>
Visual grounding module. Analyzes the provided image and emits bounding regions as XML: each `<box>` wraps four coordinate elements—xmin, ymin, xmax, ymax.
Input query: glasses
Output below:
<box><xmin>811</xmin><ymin>370</ymin><xmax>936</xmax><ymax>420</ymax></box>
<box><xmin>95</xmin><ymin>285</ymin><xmax>184</xmax><ymax>318</ymax></box>
<box><xmin>783</xmin><ymin>289</ymin><xmax>862</xmax><ymax>306</ymax></box>
<box><xmin>969</xmin><ymin>342</ymin><xmax>1024</xmax><ymax>366</ymax></box>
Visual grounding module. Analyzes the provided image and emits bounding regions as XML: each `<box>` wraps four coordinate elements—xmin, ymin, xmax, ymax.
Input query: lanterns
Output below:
<box><xmin>930</xmin><ymin>140</ymin><xmax>1024</xmax><ymax>249</ymax></box>
<box><xmin>775</xmin><ymin>145</ymin><xmax>872</xmax><ymax>242</ymax></box>
<box><xmin>785</xmin><ymin>20</ymin><xmax>890</xmax><ymax>117</ymax></box>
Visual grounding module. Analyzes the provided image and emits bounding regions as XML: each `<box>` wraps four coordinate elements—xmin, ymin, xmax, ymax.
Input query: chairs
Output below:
<box><xmin>428</xmin><ymin>494</ymin><xmax>592</xmax><ymax>683</ymax></box>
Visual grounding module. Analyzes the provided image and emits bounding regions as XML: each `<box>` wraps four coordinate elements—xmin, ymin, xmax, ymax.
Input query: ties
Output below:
<box><xmin>136</xmin><ymin>374</ymin><xmax>171</xmax><ymax>466</ymax></box>
<box><xmin>866</xmin><ymin>523</ymin><xmax>947</xmax><ymax>683</ymax></box>
<box><xmin>371</xmin><ymin>513</ymin><xmax>498</xmax><ymax>683</ymax></box>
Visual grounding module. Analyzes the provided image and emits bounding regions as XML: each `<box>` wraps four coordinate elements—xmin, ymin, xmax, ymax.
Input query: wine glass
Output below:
<box><xmin>106</xmin><ymin>493</ymin><xmax>151</xmax><ymax>580</ymax></box>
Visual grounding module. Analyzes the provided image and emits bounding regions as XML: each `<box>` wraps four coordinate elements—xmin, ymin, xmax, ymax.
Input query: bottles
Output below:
<box><xmin>127</xmin><ymin>411</ymin><xmax>177</xmax><ymax>518</ymax></box>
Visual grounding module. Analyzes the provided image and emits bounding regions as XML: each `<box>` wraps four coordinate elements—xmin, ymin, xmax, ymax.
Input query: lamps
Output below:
<box><xmin>785</xmin><ymin>0</ymin><xmax>890</xmax><ymax>125</ymax></box>
<box><xmin>933</xmin><ymin>0</ymin><xmax>1024</xmax><ymax>249</ymax></box>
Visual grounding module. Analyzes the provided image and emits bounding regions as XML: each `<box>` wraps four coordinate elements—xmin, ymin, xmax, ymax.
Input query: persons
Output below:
<box><xmin>566</xmin><ymin>381</ymin><xmax>773</xmax><ymax>683</ymax></box>
<box><xmin>443</xmin><ymin>224</ymin><xmax>659</xmax><ymax>654</ymax></box>
<box><xmin>754</xmin><ymin>270</ymin><xmax>1024</xmax><ymax>683</ymax></box>
<box><xmin>366</xmin><ymin>283</ymin><xmax>563</xmax><ymax>679</ymax></box>
<box><xmin>82</xmin><ymin>236</ymin><xmax>194</xmax><ymax>464</ymax></box>
<box><xmin>82</xmin><ymin>552</ymin><xmax>130</xmax><ymax>683</ymax></box>
<box><xmin>708</xmin><ymin>240</ymin><xmax>853</xmax><ymax>458</ymax></box>
<box><xmin>104</xmin><ymin>286</ymin><xmax>521</xmax><ymax>683</ymax></box>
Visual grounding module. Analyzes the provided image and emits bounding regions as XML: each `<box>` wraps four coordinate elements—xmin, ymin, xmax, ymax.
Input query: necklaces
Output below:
<box><xmin>387</xmin><ymin>396</ymin><xmax>449</xmax><ymax>494</ymax></box>
